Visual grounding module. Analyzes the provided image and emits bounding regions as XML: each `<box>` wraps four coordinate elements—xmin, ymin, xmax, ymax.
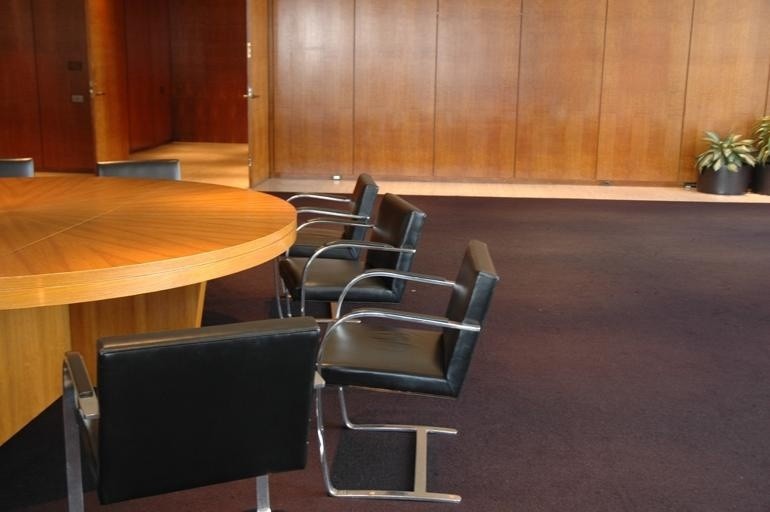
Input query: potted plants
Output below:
<box><xmin>693</xmin><ymin>131</ymin><xmax>757</xmax><ymax>195</ymax></box>
<box><xmin>752</xmin><ymin>117</ymin><xmax>770</xmax><ymax>196</ymax></box>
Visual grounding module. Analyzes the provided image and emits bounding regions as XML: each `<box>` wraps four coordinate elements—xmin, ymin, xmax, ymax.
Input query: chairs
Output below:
<box><xmin>281</xmin><ymin>174</ymin><xmax>378</xmax><ymax>295</ymax></box>
<box><xmin>278</xmin><ymin>193</ymin><xmax>426</xmax><ymax>390</ymax></box>
<box><xmin>95</xmin><ymin>160</ymin><xmax>184</xmax><ymax>183</ymax></box>
<box><xmin>0</xmin><ymin>155</ymin><xmax>35</xmax><ymax>177</ymax></box>
<box><xmin>60</xmin><ymin>316</ymin><xmax>321</xmax><ymax>512</ymax></box>
<box><xmin>316</xmin><ymin>239</ymin><xmax>499</xmax><ymax>504</ymax></box>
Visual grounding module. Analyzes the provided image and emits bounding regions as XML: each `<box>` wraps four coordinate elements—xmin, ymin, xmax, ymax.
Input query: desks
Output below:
<box><xmin>0</xmin><ymin>176</ymin><xmax>298</xmax><ymax>511</ymax></box>
